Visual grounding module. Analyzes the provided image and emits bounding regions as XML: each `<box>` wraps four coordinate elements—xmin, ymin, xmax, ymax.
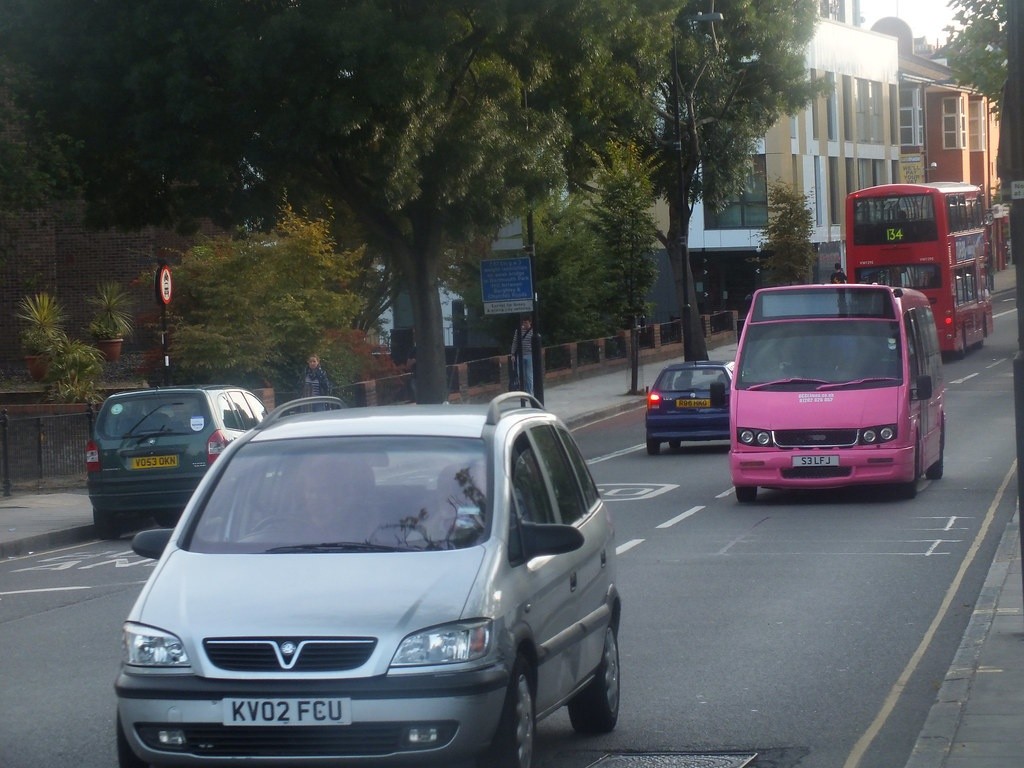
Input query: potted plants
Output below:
<box><xmin>14</xmin><ymin>289</ymin><xmax>67</xmax><ymax>378</ymax></box>
<box><xmin>87</xmin><ymin>280</ymin><xmax>133</xmax><ymax>362</ymax></box>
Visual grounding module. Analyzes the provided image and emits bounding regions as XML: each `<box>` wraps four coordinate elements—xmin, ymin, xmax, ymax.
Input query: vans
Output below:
<box><xmin>87</xmin><ymin>386</ymin><xmax>268</xmax><ymax>539</ymax></box>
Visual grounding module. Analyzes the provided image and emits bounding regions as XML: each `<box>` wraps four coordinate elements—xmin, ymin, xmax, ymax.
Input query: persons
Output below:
<box><xmin>830</xmin><ymin>263</ymin><xmax>847</xmax><ymax>284</ymax></box>
<box><xmin>510</xmin><ymin>314</ymin><xmax>534</xmax><ymax>400</ymax></box>
<box><xmin>406</xmin><ymin>463</ymin><xmax>482</xmax><ymax>543</ymax></box>
<box><xmin>297</xmin><ymin>353</ymin><xmax>332</xmax><ymax>413</ymax></box>
<box><xmin>897</xmin><ymin>210</ymin><xmax>906</xmax><ymax>221</ymax></box>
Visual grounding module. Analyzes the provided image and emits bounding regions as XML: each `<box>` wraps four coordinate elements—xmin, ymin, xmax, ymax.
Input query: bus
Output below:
<box><xmin>731</xmin><ymin>282</ymin><xmax>945</xmax><ymax>504</ymax></box>
<box><xmin>844</xmin><ymin>183</ymin><xmax>996</xmax><ymax>360</ymax></box>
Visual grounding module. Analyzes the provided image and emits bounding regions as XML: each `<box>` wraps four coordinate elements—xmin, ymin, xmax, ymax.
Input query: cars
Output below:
<box><xmin>644</xmin><ymin>360</ymin><xmax>735</xmax><ymax>454</ymax></box>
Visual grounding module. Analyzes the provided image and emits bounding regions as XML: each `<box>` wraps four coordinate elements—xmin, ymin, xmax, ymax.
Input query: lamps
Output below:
<box><xmin>757</xmin><ymin>241</ymin><xmax>763</xmax><ymax>251</ymax></box>
<box><xmin>923</xmin><ymin>160</ymin><xmax>938</xmax><ymax>172</ymax></box>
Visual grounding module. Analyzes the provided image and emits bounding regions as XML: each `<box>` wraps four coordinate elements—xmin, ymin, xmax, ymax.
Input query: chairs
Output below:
<box><xmin>411</xmin><ymin>461</ymin><xmax>483</xmax><ymax>539</ymax></box>
<box><xmin>675</xmin><ymin>375</ymin><xmax>693</xmax><ymax>391</ymax></box>
<box><xmin>717</xmin><ymin>374</ymin><xmax>729</xmax><ymax>389</ymax></box>
<box><xmin>291</xmin><ymin>449</ymin><xmax>377</xmax><ymax>539</ymax></box>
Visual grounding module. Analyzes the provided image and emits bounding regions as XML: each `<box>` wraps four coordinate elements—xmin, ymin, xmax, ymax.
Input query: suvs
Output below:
<box><xmin>114</xmin><ymin>392</ymin><xmax>623</xmax><ymax>768</ymax></box>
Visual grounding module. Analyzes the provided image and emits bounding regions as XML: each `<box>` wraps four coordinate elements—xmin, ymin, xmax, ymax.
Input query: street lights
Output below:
<box><xmin>668</xmin><ymin>13</ymin><xmax>727</xmax><ymax>349</ymax></box>
